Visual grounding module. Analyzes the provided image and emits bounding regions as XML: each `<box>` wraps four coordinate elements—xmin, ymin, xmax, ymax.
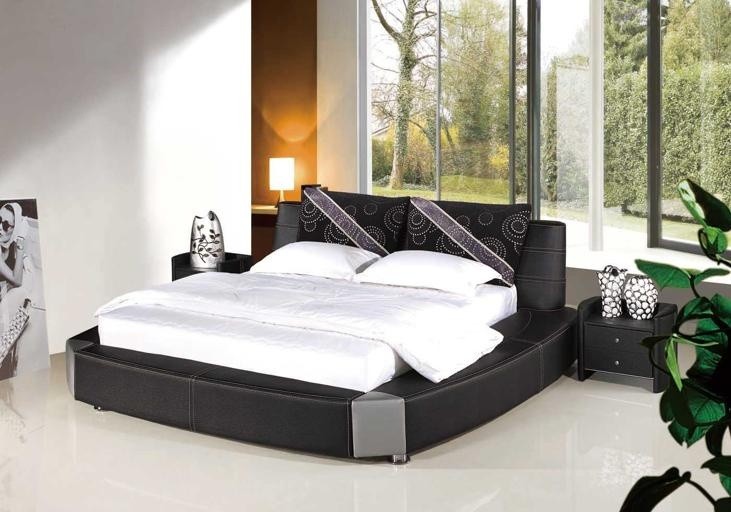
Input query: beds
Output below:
<box><xmin>64</xmin><ymin>201</ymin><xmax>578</xmax><ymax>467</ymax></box>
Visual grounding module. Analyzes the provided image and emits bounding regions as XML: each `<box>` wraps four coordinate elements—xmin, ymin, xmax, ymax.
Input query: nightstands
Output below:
<box><xmin>171</xmin><ymin>250</ymin><xmax>253</xmax><ymax>282</ymax></box>
<box><xmin>574</xmin><ymin>296</ymin><xmax>675</xmax><ymax>394</ymax></box>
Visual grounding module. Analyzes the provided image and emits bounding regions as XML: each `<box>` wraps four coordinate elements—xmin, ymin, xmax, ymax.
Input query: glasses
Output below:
<box><xmin>0</xmin><ymin>216</ymin><xmax>14</xmax><ymax>231</ymax></box>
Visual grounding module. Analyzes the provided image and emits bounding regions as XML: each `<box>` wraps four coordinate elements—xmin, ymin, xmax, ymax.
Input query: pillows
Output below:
<box><xmin>298</xmin><ymin>187</ymin><xmax>410</xmax><ymax>257</ymax></box>
<box><xmin>249</xmin><ymin>240</ymin><xmax>381</xmax><ymax>281</ymax></box>
<box><xmin>358</xmin><ymin>249</ymin><xmax>502</xmax><ymax>295</ymax></box>
<box><xmin>406</xmin><ymin>195</ymin><xmax>531</xmax><ymax>287</ymax></box>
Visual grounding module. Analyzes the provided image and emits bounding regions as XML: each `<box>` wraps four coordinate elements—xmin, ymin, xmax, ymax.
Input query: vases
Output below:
<box><xmin>623</xmin><ymin>274</ymin><xmax>659</xmax><ymax>320</ymax></box>
<box><xmin>595</xmin><ymin>264</ymin><xmax>628</xmax><ymax>317</ymax></box>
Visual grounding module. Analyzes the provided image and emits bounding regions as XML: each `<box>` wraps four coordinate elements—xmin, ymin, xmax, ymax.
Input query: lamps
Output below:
<box><xmin>269</xmin><ymin>156</ymin><xmax>296</xmax><ymax>205</ymax></box>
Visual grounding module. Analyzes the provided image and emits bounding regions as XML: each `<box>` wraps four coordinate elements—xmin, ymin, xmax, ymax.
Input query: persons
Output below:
<box><xmin>0</xmin><ymin>200</ymin><xmax>27</xmax><ymax>384</ymax></box>
